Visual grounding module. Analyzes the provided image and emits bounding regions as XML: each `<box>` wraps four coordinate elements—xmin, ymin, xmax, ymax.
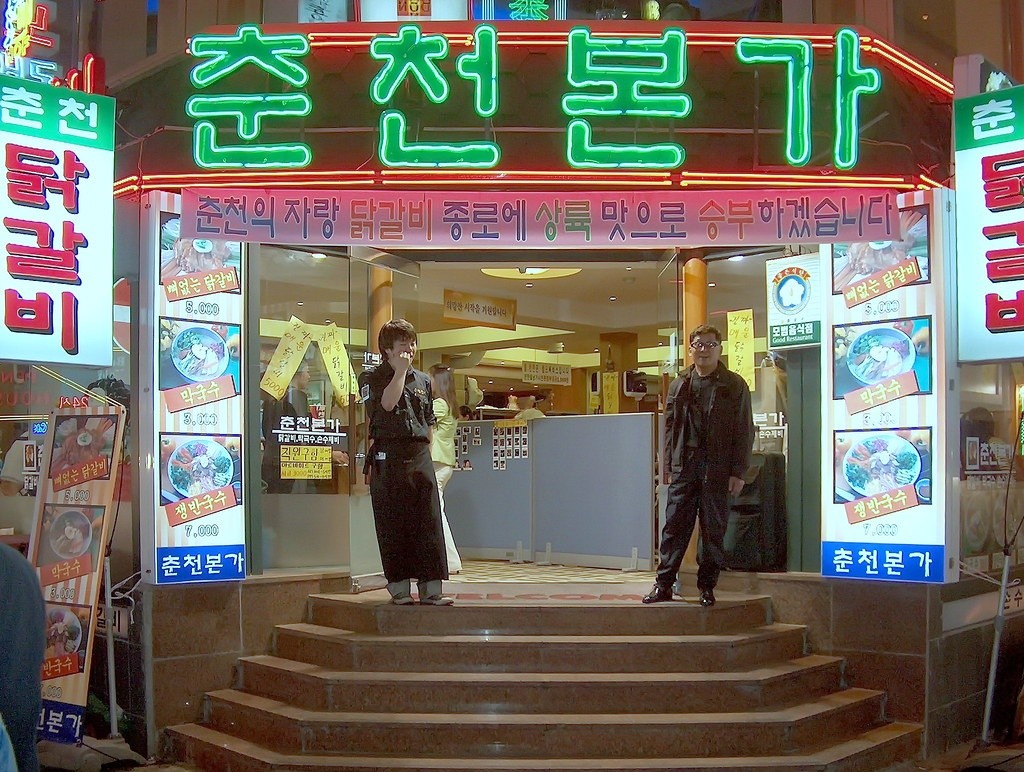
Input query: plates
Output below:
<box><xmin>45</xmin><ymin>608</ymin><xmax>81</xmax><ymax>656</ymax></box>
<box><xmin>843</xmin><ymin>434</ymin><xmax>921</xmax><ymax>497</ymax></box>
<box><xmin>50</xmin><ymin>509</ymin><xmax>92</xmax><ymax>559</ymax></box>
<box><xmin>847</xmin><ymin>326</ymin><xmax>916</xmax><ymax>387</ymax></box>
<box><xmin>167</xmin><ymin>439</ymin><xmax>233</xmax><ymax>498</ymax></box>
<box><xmin>171</xmin><ymin>326</ymin><xmax>230</xmax><ymax>383</ymax></box>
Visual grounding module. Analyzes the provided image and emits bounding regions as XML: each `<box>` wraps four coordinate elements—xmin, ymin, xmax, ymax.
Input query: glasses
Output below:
<box><xmin>691</xmin><ymin>341</ymin><xmax>721</xmax><ymax>349</ymax></box>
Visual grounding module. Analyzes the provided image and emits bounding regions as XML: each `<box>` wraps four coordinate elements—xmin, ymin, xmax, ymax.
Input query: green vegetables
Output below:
<box><xmin>176</xmin><ymin>332</ymin><xmax>203</xmax><ymax>370</ymax></box>
<box><xmin>170</xmin><ymin>445</ymin><xmax>231</xmax><ymax>489</ymax></box>
<box><xmin>854</xmin><ymin>334</ymin><xmax>880</xmax><ymax>356</ymax></box>
<box><xmin>846</xmin><ymin>440</ymin><xmax>917</xmax><ymax>489</ymax></box>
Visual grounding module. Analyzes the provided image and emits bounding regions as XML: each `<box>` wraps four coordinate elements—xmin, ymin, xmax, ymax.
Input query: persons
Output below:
<box><xmin>458</xmin><ymin>406</ymin><xmax>473</xmax><ymax>421</ymax></box>
<box><xmin>960</xmin><ymin>407</ymin><xmax>1004</xmax><ymax>481</ymax></box>
<box><xmin>642</xmin><ymin>325</ymin><xmax>755</xmax><ymax>605</ymax></box>
<box><xmin>357</xmin><ymin>318</ymin><xmax>455</xmax><ymax>605</ymax></box>
<box><xmin>0</xmin><ymin>542</ymin><xmax>46</xmax><ymax>772</ymax></box>
<box><xmin>513</xmin><ymin>393</ymin><xmax>551</xmax><ymax>419</ymax></box>
<box><xmin>427</xmin><ymin>363</ymin><xmax>462</xmax><ymax>574</ymax></box>
<box><xmin>261</xmin><ymin>358</ymin><xmax>349</xmax><ymax>494</ymax></box>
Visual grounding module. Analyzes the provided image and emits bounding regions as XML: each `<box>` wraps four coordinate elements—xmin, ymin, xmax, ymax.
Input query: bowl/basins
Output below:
<box><xmin>77</xmin><ymin>649</ymin><xmax>86</xmax><ymax>669</ymax></box>
<box><xmin>915</xmin><ymin>479</ymin><xmax>930</xmax><ymax>501</ymax></box>
<box><xmin>232</xmin><ymin>481</ymin><xmax>241</xmax><ymax>502</ymax></box>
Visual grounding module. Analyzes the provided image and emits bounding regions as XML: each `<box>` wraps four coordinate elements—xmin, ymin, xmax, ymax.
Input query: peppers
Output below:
<box><xmin>836</xmin><ymin>436</ymin><xmax>851</xmax><ymax>453</ymax></box>
<box><xmin>161</xmin><ymin>439</ymin><xmax>175</xmax><ymax>455</ymax></box>
<box><xmin>893</xmin><ymin>319</ymin><xmax>930</xmax><ymax>355</ymax></box>
<box><xmin>211</xmin><ymin>325</ymin><xmax>239</xmax><ymax>359</ymax></box>
<box><xmin>898</xmin><ymin>429</ymin><xmax>929</xmax><ymax>451</ymax></box>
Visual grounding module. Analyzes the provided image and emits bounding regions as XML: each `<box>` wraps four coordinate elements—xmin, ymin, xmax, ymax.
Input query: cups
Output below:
<box><xmin>310</xmin><ymin>405</ymin><xmax>321</xmax><ymax>420</ymax></box>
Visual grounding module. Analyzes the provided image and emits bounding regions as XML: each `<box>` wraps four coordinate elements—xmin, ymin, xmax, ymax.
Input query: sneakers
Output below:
<box><xmin>423</xmin><ymin>594</ymin><xmax>454</xmax><ymax>606</ymax></box>
<box><xmin>393</xmin><ymin>592</ymin><xmax>415</xmax><ymax>605</ymax></box>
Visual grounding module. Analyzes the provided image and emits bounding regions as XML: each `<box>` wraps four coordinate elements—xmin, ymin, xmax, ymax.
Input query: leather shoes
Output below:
<box><xmin>700</xmin><ymin>589</ymin><xmax>716</xmax><ymax>606</ymax></box>
<box><xmin>643</xmin><ymin>587</ymin><xmax>673</xmax><ymax>603</ymax></box>
